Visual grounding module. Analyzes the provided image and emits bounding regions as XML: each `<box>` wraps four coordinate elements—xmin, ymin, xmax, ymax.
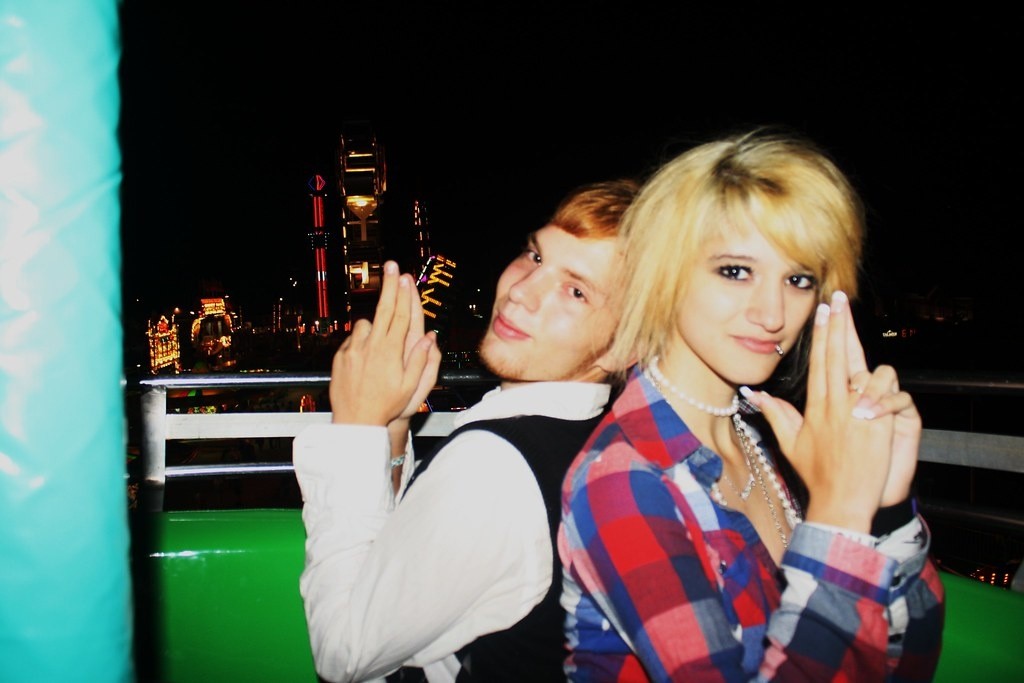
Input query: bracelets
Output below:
<box><xmin>871</xmin><ymin>500</ymin><xmax>917</xmax><ymax>536</ymax></box>
<box><xmin>391</xmin><ymin>455</ymin><xmax>405</xmax><ymax>468</ymax></box>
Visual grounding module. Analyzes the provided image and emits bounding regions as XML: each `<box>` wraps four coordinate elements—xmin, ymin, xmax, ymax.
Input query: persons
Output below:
<box><xmin>292</xmin><ymin>180</ymin><xmax>641</xmax><ymax>683</ymax></box>
<box><xmin>558</xmin><ymin>134</ymin><xmax>945</xmax><ymax>683</ymax></box>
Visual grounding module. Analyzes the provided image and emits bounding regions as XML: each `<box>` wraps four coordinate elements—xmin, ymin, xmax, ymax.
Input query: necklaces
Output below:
<box><xmin>645</xmin><ymin>355</ymin><xmax>801</xmax><ymax>550</ymax></box>
